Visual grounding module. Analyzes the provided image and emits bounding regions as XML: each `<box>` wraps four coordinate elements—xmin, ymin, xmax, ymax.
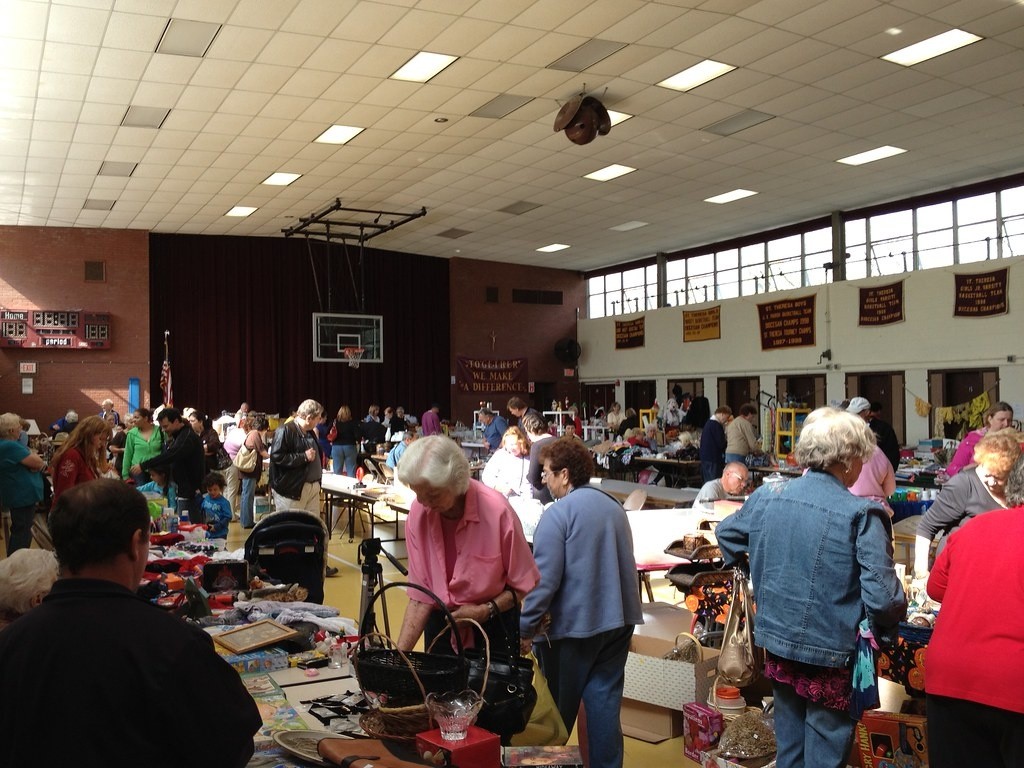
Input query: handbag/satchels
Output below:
<box><xmin>327</xmin><ymin>419</ymin><xmax>337</xmax><ymax>442</ymax></box>
<box><xmin>466</xmin><ymin>587</ymin><xmax>537</xmax><ymax>734</ymax></box>
<box><xmin>209</xmin><ymin>445</ymin><xmax>233</xmax><ymax>470</ymax></box>
<box><xmin>234</xmin><ymin>430</ymin><xmax>257</xmax><ymax>473</ymax></box>
<box><xmin>159</xmin><ymin>426</ymin><xmax>167</xmax><ymax>456</ymax></box>
<box><xmin>716</xmin><ymin>567</ymin><xmax>761</xmax><ymax>687</ymax></box>
<box><xmin>510</xmin><ymin>652</ymin><xmax>569</xmax><ymax>747</ymax></box>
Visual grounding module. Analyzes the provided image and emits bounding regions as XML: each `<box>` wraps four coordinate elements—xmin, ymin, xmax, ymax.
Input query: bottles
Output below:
<box><xmin>328</xmin><ymin>645</ymin><xmax>343</xmax><ymax>668</ymax></box>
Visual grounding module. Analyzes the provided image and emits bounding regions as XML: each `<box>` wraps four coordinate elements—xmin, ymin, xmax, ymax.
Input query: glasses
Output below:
<box><xmin>541</xmin><ymin>470</ymin><xmax>561</xmax><ymax>479</ymax></box>
<box><xmin>732</xmin><ymin>473</ymin><xmax>747</xmax><ymax>484</ymax></box>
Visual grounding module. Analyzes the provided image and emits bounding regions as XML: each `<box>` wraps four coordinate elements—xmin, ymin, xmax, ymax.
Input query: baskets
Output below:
<box><xmin>349</xmin><ymin>582</ymin><xmax>472</xmax><ymax>701</ymax></box>
<box><xmin>347</xmin><ymin>617</ymin><xmax>489</xmax><ymax>734</ymax></box>
<box><xmin>360</xmin><ymin>709</ymin><xmax>425</xmax><ymax>765</ymax></box>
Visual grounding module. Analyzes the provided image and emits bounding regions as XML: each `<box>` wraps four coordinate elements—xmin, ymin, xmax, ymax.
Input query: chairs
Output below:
<box><xmin>243</xmin><ymin>505</ymin><xmax>332</xmax><ymax>613</ymax></box>
<box><xmin>363</xmin><ymin>458</ymin><xmax>385</xmax><ymax>483</ymax></box>
<box><xmin>380</xmin><ymin>461</ymin><xmax>396</xmax><ymax>486</ymax></box>
<box><xmin>332</xmin><ymin>474</ymin><xmax>374</xmax><ymax>540</ymax></box>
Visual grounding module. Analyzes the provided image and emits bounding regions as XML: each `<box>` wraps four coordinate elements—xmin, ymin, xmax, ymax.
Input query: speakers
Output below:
<box><xmin>554</xmin><ymin>338</ymin><xmax>578</xmax><ymax>362</ymax></box>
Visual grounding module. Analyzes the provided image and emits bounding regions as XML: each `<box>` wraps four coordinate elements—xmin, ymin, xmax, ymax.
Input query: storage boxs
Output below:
<box><xmin>855</xmin><ymin>713</ymin><xmax>929</xmax><ymax>768</ymax></box>
<box><xmin>918</xmin><ymin>437</ymin><xmax>943</xmax><ymax>451</ymax></box>
<box><xmin>900</xmin><ymin>448</ymin><xmax>914</xmax><ymax>457</ymax></box>
<box><xmin>202</xmin><ymin>561</ymin><xmax>249</xmax><ymax>591</ymax></box>
<box><xmin>714</xmin><ymin>499</ymin><xmax>744</xmax><ymax>514</ymax></box>
<box><xmin>414</xmin><ymin>724</ymin><xmax>501</xmax><ymax>768</ymax></box>
<box><xmin>622</xmin><ymin>632</ymin><xmax>724</xmax><ymax>712</ymax></box>
<box><xmin>683</xmin><ymin>700</ymin><xmax>725</xmax><ymax>763</ymax></box>
<box><xmin>913</xmin><ymin>449</ymin><xmax>935</xmax><ymax>459</ymax></box>
<box><xmin>620</xmin><ymin>698</ymin><xmax>682</xmax><ymax>743</ymax></box>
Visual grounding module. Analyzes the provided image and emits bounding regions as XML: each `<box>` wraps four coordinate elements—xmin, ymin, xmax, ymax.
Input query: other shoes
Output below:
<box><xmin>326</xmin><ymin>566</ymin><xmax>338</xmax><ymax>576</ymax></box>
<box><xmin>244</xmin><ymin>522</ymin><xmax>256</xmax><ymax>529</ymax></box>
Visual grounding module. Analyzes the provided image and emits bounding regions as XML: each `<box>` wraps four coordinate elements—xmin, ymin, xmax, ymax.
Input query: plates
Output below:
<box><xmin>273</xmin><ymin>730</ymin><xmax>356</xmax><ymax>766</ymax></box>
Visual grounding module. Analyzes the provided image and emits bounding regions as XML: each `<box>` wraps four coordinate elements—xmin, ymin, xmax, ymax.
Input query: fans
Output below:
<box><xmin>554</xmin><ymin>337</ymin><xmax>582</xmax><ymax>369</ymax></box>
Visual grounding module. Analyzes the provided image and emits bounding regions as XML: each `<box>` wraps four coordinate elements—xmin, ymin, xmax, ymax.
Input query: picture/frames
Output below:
<box><xmin>211</xmin><ymin>618</ymin><xmax>301</xmax><ymax>653</ymax></box>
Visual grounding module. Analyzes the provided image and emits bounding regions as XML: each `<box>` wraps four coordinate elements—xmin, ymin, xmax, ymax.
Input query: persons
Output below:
<box><xmin>522</xmin><ymin>413</ymin><xmax>558</xmax><ymax>505</ymax></box>
<box><xmin>0</xmin><ymin>477</ymin><xmax>263</xmax><ymax>768</ymax></box>
<box><xmin>396</xmin><ymin>435</ymin><xmax>542</xmax><ymax>657</ymax></box>
<box><xmin>724</xmin><ymin>403</ymin><xmax>762</xmax><ymax>464</ymax></box>
<box><xmin>699</xmin><ymin>405</ymin><xmax>732</xmax><ymax>484</ymax></box>
<box><xmin>801</xmin><ymin>406</ymin><xmax>896</xmax><ymax>558</ymax></box>
<box><xmin>481</xmin><ymin>426</ymin><xmax>544</xmax><ymax>554</ymax></box>
<box><xmin>0</xmin><ymin>548</ymin><xmax>60</xmax><ymax>630</ymax></box>
<box><xmin>0</xmin><ymin>413</ymin><xmax>44</xmax><ymax>558</ymax></box>
<box><xmin>519</xmin><ymin>435</ymin><xmax>645</xmax><ymax>768</ymax></box>
<box><xmin>269</xmin><ymin>399</ymin><xmax>338</xmax><ymax>576</ymax></box>
<box><xmin>945</xmin><ymin>402</ymin><xmax>1013</xmax><ymax>478</ymax></box>
<box><xmin>0</xmin><ymin>392</ymin><xmax>700</xmax><ymax>555</ymax></box>
<box><xmin>692</xmin><ymin>461</ymin><xmax>748</xmax><ymax>509</ymax></box>
<box><xmin>714</xmin><ymin>412</ymin><xmax>908</xmax><ymax>768</ymax></box>
<box><xmin>201</xmin><ymin>473</ymin><xmax>232</xmax><ymax>539</ymax></box>
<box><xmin>845</xmin><ymin>397</ymin><xmax>900</xmax><ymax>473</ymax></box>
<box><xmin>924</xmin><ymin>450</ymin><xmax>1024</xmax><ymax>768</ymax></box>
<box><xmin>914</xmin><ymin>435</ymin><xmax>1021</xmax><ymax>580</ymax></box>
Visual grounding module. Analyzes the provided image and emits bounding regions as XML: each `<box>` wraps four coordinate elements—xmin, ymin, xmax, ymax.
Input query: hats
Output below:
<box><xmin>846</xmin><ymin>396</ymin><xmax>871</xmax><ymax>414</ymax></box>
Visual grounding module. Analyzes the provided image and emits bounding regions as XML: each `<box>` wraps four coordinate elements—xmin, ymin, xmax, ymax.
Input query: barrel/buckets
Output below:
<box><xmin>706</xmin><ymin>676</ymin><xmax>746</xmax><ymax>717</ymax></box>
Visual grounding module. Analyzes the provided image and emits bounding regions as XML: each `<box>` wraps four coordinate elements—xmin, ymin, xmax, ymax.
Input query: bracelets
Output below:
<box><xmin>487</xmin><ymin>602</ymin><xmax>494</xmax><ymax>619</ymax></box>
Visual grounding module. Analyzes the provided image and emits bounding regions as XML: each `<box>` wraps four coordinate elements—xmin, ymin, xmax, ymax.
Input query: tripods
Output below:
<box><xmin>357</xmin><ymin>537</ymin><xmax>408</xmax><ymax>652</ymax></box>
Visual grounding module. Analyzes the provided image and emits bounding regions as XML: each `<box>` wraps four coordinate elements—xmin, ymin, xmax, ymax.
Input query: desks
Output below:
<box><xmin>473</xmin><ymin>410</ymin><xmax>499</xmax><ymax>439</ymax></box>
<box><xmin>0</xmin><ymin>426</ymin><xmax>1024</xmax><ymax>768</ymax></box>
<box><xmin>543</xmin><ymin>411</ymin><xmax>575</xmax><ymax>436</ymax></box>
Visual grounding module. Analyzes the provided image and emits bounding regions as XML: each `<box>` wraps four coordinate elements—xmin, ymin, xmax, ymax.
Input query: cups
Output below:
<box><xmin>425</xmin><ymin>690</ymin><xmax>482</xmax><ymax>740</ymax></box>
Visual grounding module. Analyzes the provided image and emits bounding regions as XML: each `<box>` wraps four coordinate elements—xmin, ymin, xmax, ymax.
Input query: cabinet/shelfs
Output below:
<box><xmin>640</xmin><ymin>409</ymin><xmax>658</xmax><ymax>432</ymax></box>
<box><xmin>776</xmin><ymin>407</ymin><xmax>812</xmax><ymax>457</ymax></box>
